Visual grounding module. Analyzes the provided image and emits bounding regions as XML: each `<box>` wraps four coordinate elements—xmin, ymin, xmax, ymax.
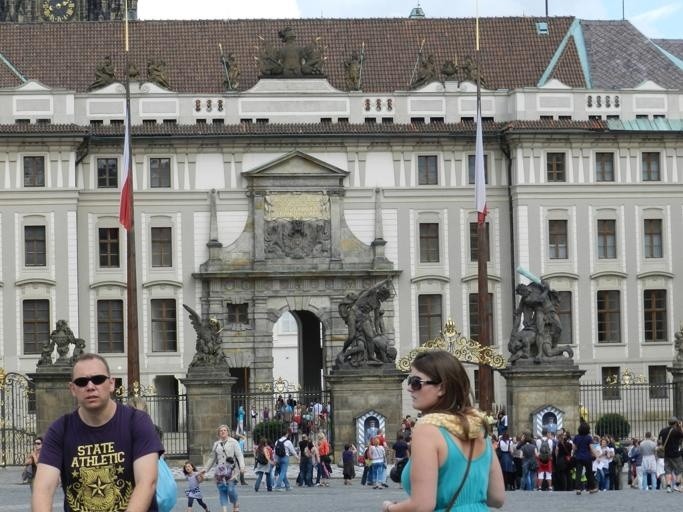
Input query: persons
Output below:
<box><xmin>343</xmin><ymin>49</ymin><xmax>368</xmax><ymax>91</ymax></box>
<box><xmin>199</xmin><ymin>424</ymin><xmax>246</xmax><ymax>512</ymax></box>
<box><xmin>455</xmin><ymin>55</ymin><xmax>486</xmax><ymax>87</ymax></box>
<box><xmin>340</xmin><ymin>334</ymin><xmax>397</xmax><ymax>363</ymax></box>
<box><xmin>381</xmin><ymin>350</ymin><xmax>506</xmax><ymax>512</ymax></box>
<box><xmin>489</xmin><ymin>417</ymin><xmax>683</xmax><ymax>493</ymax></box>
<box><xmin>182</xmin><ymin>461</ymin><xmax>211</xmax><ymax>512</ymax></box>
<box><xmin>126</xmin><ymin>396</ymin><xmax>178</xmax><ymax>512</ymax></box>
<box><xmin>508</xmin><ymin>330</ymin><xmax>574</xmax><ymax>359</ymax></box>
<box><xmin>578</xmin><ymin>403</ymin><xmax>588</xmax><ymax>421</ymax></box>
<box><xmin>516</xmin><ymin>278</ymin><xmax>574</xmax><ymax>363</ymax></box>
<box><xmin>25</xmin><ymin>437</ymin><xmax>43</xmax><ymax>480</ymax></box>
<box><xmin>146</xmin><ymin>61</ymin><xmax>172</xmax><ymax>90</ymax></box>
<box><xmin>85</xmin><ymin>58</ymin><xmax>116</xmax><ymax>93</ymax></box>
<box><xmin>340</xmin><ymin>278</ymin><xmax>392</xmax><ymax>361</ymax></box>
<box><xmin>234</xmin><ymin>394</ymin><xmax>421</xmax><ymax>491</ymax></box>
<box><xmin>496</xmin><ymin>407</ymin><xmax>508</xmax><ymax>434</ymax></box>
<box><xmin>220</xmin><ymin>52</ymin><xmax>241</xmax><ymax>90</ymax></box>
<box><xmin>30</xmin><ymin>352</ymin><xmax>166</xmax><ymax>512</ymax></box>
<box><xmin>406</xmin><ymin>53</ymin><xmax>437</xmax><ymax>91</ymax></box>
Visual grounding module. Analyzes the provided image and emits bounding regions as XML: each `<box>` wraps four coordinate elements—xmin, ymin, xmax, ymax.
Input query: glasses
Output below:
<box><xmin>72</xmin><ymin>375</ymin><xmax>110</xmax><ymax>386</ymax></box>
<box><xmin>407</xmin><ymin>377</ymin><xmax>434</xmax><ymax>391</ymax></box>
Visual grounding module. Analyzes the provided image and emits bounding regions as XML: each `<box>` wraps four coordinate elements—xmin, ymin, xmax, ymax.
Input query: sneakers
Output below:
<box><xmin>538</xmin><ymin>486</ymin><xmax>553</xmax><ymax>491</ymax></box>
<box><xmin>666</xmin><ymin>484</ymin><xmax>683</xmax><ymax>493</ymax></box>
<box><xmin>576</xmin><ymin>489</ymin><xmax>598</xmax><ymax>494</ymax></box>
<box><xmin>373</xmin><ymin>482</ymin><xmax>388</xmax><ymax>489</ymax></box>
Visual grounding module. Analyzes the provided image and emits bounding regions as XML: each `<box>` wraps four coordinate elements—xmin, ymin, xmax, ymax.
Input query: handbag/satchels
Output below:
<box><xmin>338</xmin><ymin>457</ymin><xmax>344</xmax><ymax>468</ymax></box>
<box><xmin>358</xmin><ymin>456</ymin><xmax>365</xmax><ymax>463</ymax></box>
<box><xmin>383</xmin><ymin>457</ymin><xmax>388</xmax><ymax>469</ymax></box>
<box><xmin>529</xmin><ymin>458</ymin><xmax>537</xmax><ymax>470</ymax></box>
<box><xmin>657</xmin><ymin>446</ymin><xmax>666</xmax><ymax>458</ymax></box>
<box><xmin>255</xmin><ymin>448</ymin><xmax>268</xmax><ymax>465</ymax></box>
<box><xmin>495</xmin><ymin>447</ymin><xmax>502</xmax><ymax>458</ymax></box>
<box><xmin>566</xmin><ymin>455</ymin><xmax>576</xmax><ymax>467</ymax></box>
<box><xmin>304</xmin><ymin>447</ymin><xmax>311</xmax><ymax>457</ymax></box>
<box><xmin>214</xmin><ymin>464</ymin><xmax>233</xmax><ymax>479</ymax></box>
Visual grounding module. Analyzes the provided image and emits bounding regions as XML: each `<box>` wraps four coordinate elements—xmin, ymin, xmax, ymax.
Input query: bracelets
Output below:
<box><xmin>387</xmin><ymin>500</ymin><xmax>398</xmax><ymax>512</ymax></box>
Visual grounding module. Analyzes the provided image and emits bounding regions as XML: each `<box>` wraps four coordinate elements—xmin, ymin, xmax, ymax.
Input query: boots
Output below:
<box><xmin>240</xmin><ymin>472</ymin><xmax>248</xmax><ymax>485</ymax></box>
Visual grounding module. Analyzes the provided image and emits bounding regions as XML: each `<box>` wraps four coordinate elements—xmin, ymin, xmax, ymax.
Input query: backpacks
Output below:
<box><xmin>275</xmin><ymin>439</ymin><xmax>288</xmax><ymax>456</ymax></box>
<box><xmin>539</xmin><ymin>438</ymin><xmax>550</xmax><ymax>464</ymax></box>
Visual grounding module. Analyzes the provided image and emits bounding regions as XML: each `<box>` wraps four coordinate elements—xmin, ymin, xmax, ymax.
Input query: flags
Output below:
<box><xmin>118</xmin><ymin>97</ymin><xmax>132</xmax><ymax>232</ymax></box>
<box><xmin>474</xmin><ymin>87</ymin><xmax>488</xmax><ymax>225</ymax></box>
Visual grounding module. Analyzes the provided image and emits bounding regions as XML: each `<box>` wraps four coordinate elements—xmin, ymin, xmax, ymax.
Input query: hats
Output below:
<box><xmin>668</xmin><ymin>416</ymin><xmax>678</xmax><ymax>423</ymax></box>
